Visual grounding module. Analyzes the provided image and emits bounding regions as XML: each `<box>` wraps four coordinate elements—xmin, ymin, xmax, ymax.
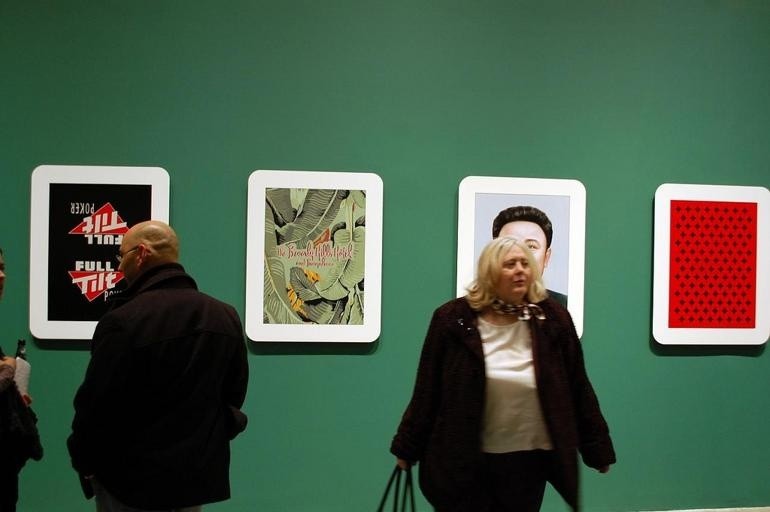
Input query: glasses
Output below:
<box><xmin>116</xmin><ymin>245</ymin><xmax>141</xmax><ymax>261</ymax></box>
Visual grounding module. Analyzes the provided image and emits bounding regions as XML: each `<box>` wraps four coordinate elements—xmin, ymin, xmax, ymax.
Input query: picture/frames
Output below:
<box><xmin>651</xmin><ymin>179</ymin><xmax>770</xmax><ymax>348</ymax></box>
<box><xmin>451</xmin><ymin>171</ymin><xmax>586</xmax><ymax>345</ymax></box>
<box><xmin>28</xmin><ymin>163</ymin><xmax>173</xmax><ymax>341</ymax></box>
<box><xmin>241</xmin><ymin>170</ymin><xmax>386</xmax><ymax>348</ymax></box>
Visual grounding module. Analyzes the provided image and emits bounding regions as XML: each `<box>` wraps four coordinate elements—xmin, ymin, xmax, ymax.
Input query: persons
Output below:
<box><xmin>490</xmin><ymin>205</ymin><xmax>568</xmax><ymax>311</ymax></box>
<box><xmin>65</xmin><ymin>218</ymin><xmax>250</xmax><ymax>509</ymax></box>
<box><xmin>388</xmin><ymin>236</ymin><xmax>617</xmax><ymax>511</ymax></box>
<box><xmin>0</xmin><ymin>249</ymin><xmax>46</xmax><ymax>512</ymax></box>
